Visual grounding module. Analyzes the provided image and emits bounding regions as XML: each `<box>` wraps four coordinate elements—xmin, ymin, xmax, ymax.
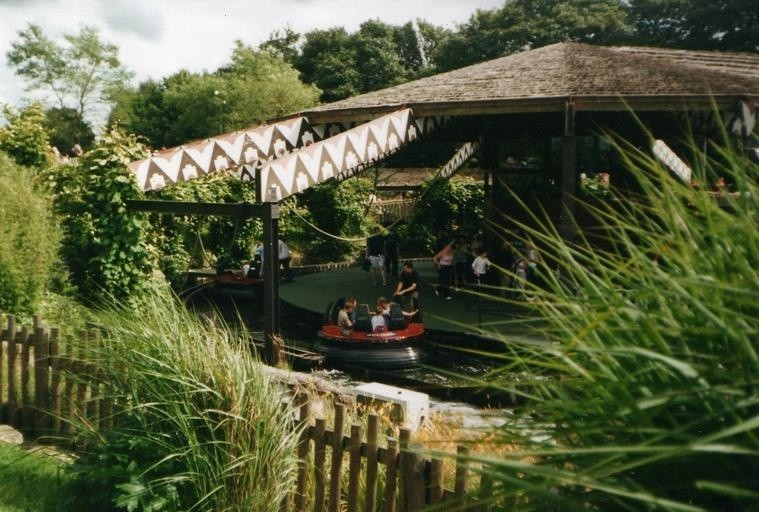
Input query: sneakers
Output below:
<box><xmin>382</xmin><ymin>281</ymin><xmax>391</xmax><ymax>286</ymax></box>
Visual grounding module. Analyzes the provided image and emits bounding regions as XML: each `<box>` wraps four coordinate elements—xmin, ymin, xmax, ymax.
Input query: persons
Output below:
<box><xmin>278</xmin><ymin>239</ymin><xmax>291</xmax><ymax>280</ymax></box>
<box><xmin>251</xmin><ymin>242</ymin><xmax>264</xmax><ymax>279</ymax></box>
<box><xmin>338</xmin><ymin>227</ymin><xmax>540</xmax><ymax>333</ymax></box>
